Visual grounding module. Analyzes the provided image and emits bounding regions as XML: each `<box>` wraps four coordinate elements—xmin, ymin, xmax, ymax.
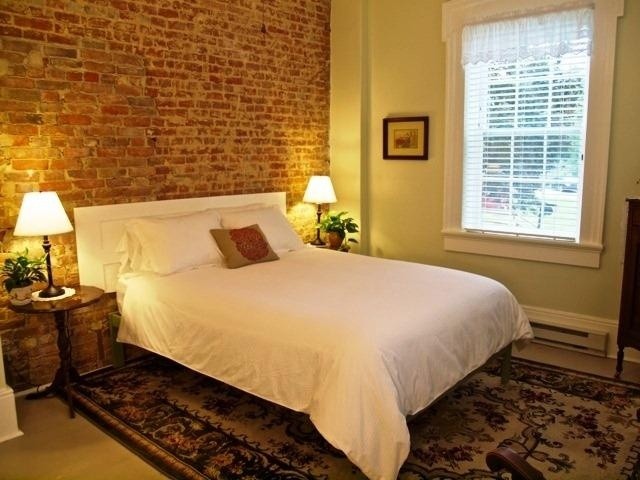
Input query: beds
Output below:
<box><xmin>73</xmin><ymin>190</ymin><xmax>532</xmax><ymax>480</ymax></box>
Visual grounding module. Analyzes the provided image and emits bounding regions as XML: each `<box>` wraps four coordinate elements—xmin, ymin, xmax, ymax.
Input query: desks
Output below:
<box><xmin>9</xmin><ymin>283</ymin><xmax>106</xmax><ymax>420</ymax></box>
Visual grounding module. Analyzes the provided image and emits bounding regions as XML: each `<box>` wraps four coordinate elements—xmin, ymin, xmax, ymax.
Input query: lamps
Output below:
<box><xmin>302</xmin><ymin>175</ymin><xmax>338</xmax><ymax>246</ymax></box>
<box><xmin>13</xmin><ymin>191</ymin><xmax>75</xmax><ymax>298</ymax></box>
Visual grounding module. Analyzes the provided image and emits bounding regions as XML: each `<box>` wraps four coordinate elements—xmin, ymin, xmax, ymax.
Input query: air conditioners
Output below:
<box><xmin>527</xmin><ymin>319</ymin><xmax>610</xmax><ymax>356</ymax></box>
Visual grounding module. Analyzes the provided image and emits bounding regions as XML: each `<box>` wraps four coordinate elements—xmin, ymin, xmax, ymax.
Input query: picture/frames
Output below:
<box><xmin>382</xmin><ymin>116</ymin><xmax>430</xmax><ymax>161</ymax></box>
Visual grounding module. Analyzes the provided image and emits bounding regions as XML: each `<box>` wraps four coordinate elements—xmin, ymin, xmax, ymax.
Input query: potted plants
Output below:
<box><xmin>314</xmin><ymin>212</ymin><xmax>359</xmax><ymax>249</ymax></box>
<box><xmin>2</xmin><ymin>247</ymin><xmax>49</xmax><ymax>306</ymax></box>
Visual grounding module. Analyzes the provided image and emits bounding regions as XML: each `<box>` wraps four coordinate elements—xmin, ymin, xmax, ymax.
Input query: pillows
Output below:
<box><xmin>208</xmin><ymin>224</ymin><xmax>280</xmax><ymax>269</ymax></box>
<box><xmin>132</xmin><ymin>210</ymin><xmax>225</xmax><ymax>273</ymax></box>
<box><xmin>220</xmin><ymin>205</ymin><xmax>305</xmax><ymax>252</ymax></box>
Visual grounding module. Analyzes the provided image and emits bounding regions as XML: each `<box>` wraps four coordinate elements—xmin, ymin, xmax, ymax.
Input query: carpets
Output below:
<box><xmin>54</xmin><ymin>349</ymin><xmax>640</xmax><ymax>480</ymax></box>
